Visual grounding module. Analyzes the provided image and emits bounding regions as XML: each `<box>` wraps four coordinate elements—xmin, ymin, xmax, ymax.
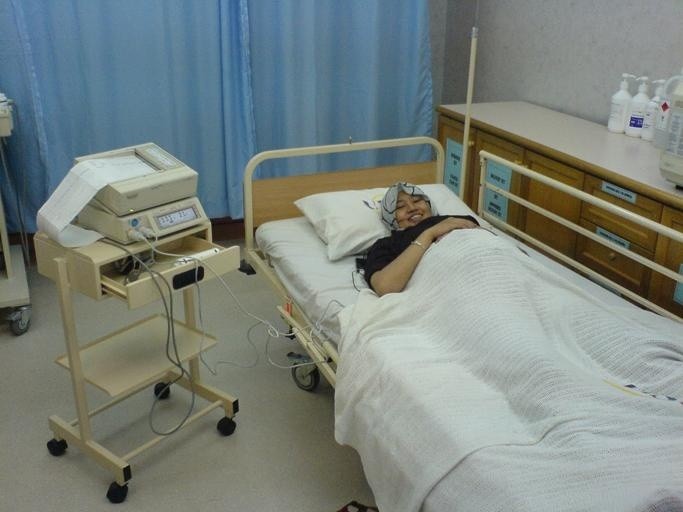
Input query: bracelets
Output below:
<box><xmin>409</xmin><ymin>241</ymin><xmax>426</xmax><ymax>251</ymax></box>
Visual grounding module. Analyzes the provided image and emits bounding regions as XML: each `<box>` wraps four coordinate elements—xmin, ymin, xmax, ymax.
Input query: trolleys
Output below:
<box><xmin>27</xmin><ymin>226</ymin><xmax>241</xmax><ymax>505</ymax></box>
<box><xmin>0</xmin><ymin>96</ymin><xmax>33</xmax><ymax>333</ymax></box>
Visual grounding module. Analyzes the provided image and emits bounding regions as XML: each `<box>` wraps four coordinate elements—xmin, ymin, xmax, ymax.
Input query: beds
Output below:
<box><xmin>238</xmin><ymin>137</ymin><xmax>683</xmax><ymax>512</ymax></box>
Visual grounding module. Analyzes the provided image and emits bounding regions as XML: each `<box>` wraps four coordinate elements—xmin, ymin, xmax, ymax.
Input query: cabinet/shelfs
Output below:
<box><xmin>472</xmin><ymin>130</ymin><xmax>585</xmax><ymax>263</ymax></box>
<box><xmin>437</xmin><ymin>116</ymin><xmax>477</xmax><ymax>214</ymax></box>
<box><xmin>574</xmin><ymin>171</ymin><xmax>683</xmax><ymax>321</ymax></box>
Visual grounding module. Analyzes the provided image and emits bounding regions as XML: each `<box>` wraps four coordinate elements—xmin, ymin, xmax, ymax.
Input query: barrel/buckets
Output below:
<box><xmin>653</xmin><ymin>75</ymin><xmax>683</xmax><ymax>149</ymax></box>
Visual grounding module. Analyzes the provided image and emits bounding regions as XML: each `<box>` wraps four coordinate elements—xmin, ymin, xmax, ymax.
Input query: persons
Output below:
<box><xmin>363</xmin><ymin>180</ymin><xmax>480</xmax><ymax>297</ymax></box>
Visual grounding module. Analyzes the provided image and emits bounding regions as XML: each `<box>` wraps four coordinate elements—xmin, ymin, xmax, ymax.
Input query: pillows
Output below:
<box><xmin>294</xmin><ymin>184</ymin><xmax>492</xmax><ymax>261</ymax></box>
